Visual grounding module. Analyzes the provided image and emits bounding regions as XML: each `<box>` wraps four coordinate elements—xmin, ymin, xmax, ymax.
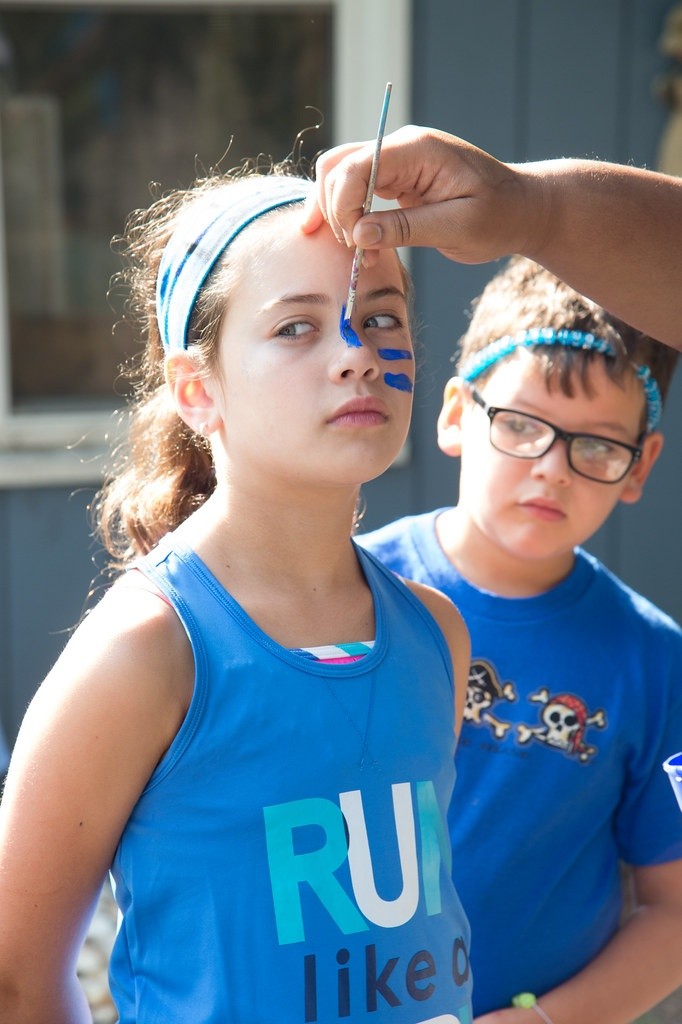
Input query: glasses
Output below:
<box><xmin>472</xmin><ymin>389</ymin><xmax>642</xmax><ymax>484</ymax></box>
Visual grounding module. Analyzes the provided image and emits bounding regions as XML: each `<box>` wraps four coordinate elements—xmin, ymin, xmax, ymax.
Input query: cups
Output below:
<box><xmin>662</xmin><ymin>751</ymin><xmax>682</xmax><ymax>813</ymax></box>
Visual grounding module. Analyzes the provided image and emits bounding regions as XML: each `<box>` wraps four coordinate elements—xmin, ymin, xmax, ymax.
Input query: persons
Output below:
<box><xmin>351</xmin><ymin>263</ymin><xmax>682</xmax><ymax>1024</ymax></box>
<box><xmin>0</xmin><ymin>177</ymin><xmax>472</xmax><ymax>1024</ymax></box>
<box><xmin>301</xmin><ymin>125</ymin><xmax>681</xmax><ymax>355</ymax></box>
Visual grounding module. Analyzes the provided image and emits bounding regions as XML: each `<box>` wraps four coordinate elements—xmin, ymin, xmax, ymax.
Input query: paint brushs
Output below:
<box><xmin>343</xmin><ymin>82</ymin><xmax>394</xmax><ymax>328</ymax></box>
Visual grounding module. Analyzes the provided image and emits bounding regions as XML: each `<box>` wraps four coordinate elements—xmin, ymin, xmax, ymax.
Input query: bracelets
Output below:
<box><xmin>514</xmin><ymin>993</ymin><xmax>553</xmax><ymax>1024</ymax></box>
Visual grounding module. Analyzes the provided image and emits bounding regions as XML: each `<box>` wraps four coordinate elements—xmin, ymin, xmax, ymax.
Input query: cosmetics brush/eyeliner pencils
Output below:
<box><xmin>343</xmin><ymin>82</ymin><xmax>392</xmax><ymax>328</ymax></box>
